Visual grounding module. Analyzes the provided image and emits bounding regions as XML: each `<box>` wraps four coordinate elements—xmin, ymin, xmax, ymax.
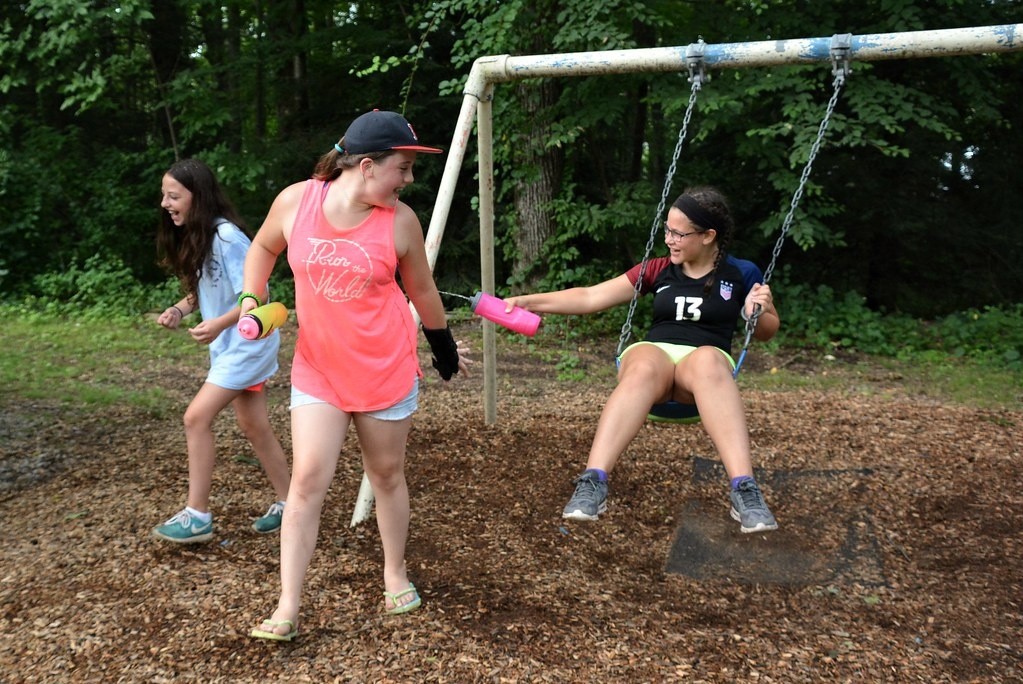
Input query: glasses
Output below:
<box><xmin>664</xmin><ymin>221</ymin><xmax>705</xmax><ymax>242</ymax></box>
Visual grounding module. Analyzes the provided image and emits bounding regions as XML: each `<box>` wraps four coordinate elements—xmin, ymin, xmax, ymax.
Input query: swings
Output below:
<box><xmin>614</xmin><ymin>32</ymin><xmax>853</xmax><ymax>425</ymax></box>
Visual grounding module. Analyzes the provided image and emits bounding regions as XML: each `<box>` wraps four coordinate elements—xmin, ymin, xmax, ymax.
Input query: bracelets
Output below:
<box><xmin>171</xmin><ymin>306</ymin><xmax>183</xmax><ymax>318</ymax></box>
<box><xmin>238</xmin><ymin>292</ymin><xmax>262</xmax><ymax>307</ymax></box>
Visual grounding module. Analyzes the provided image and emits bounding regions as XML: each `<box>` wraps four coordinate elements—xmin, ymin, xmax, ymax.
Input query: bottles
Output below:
<box><xmin>468</xmin><ymin>292</ymin><xmax>542</xmax><ymax>337</ymax></box>
<box><xmin>238</xmin><ymin>302</ymin><xmax>288</xmax><ymax>340</ymax></box>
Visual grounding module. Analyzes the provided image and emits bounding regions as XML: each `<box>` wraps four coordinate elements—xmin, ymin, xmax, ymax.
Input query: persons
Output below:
<box><xmin>152</xmin><ymin>160</ymin><xmax>290</xmax><ymax>542</ymax></box>
<box><xmin>504</xmin><ymin>188</ymin><xmax>779</xmax><ymax>532</ymax></box>
<box><xmin>239</xmin><ymin>109</ymin><xmax>469</xmax><ymax>641</ymax></box>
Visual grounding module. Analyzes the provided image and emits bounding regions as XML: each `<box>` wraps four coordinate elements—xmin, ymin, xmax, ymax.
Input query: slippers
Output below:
<box><xmin>383</xmin><ymin>582</ymin><xmax>422</xmax><ymax>615</ymax></box>
<box><xmin>251</xmin><ymin>619</ymin><xmax>298</xmax><ymax>642</ymax></box>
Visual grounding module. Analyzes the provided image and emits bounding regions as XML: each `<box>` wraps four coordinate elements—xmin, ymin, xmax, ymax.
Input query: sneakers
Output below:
<box><xmin>562</xmin><ymin>473</ymin><xmax>608</xmax><ymax>521</ymax></box>
<box><xmin>153</xmin><ymin>509</ymin><xmax>214</xmax><ymax>543</ymax></box>
<box><xmin>729</xmin><ymin>476</ymin><xmax>779</xmax><ymax>533</ymax></box>
<box><xmin>251</xmin><ymin>501</ymin><xmax>286</xmax><ymax>535</ymax></box>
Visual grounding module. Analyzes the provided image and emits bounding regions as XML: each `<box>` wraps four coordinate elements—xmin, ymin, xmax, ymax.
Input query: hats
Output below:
<box><xmin>344</xmin><ymin>109</ymin><xmax>443</xmax><ymax>154</ymax></box>
